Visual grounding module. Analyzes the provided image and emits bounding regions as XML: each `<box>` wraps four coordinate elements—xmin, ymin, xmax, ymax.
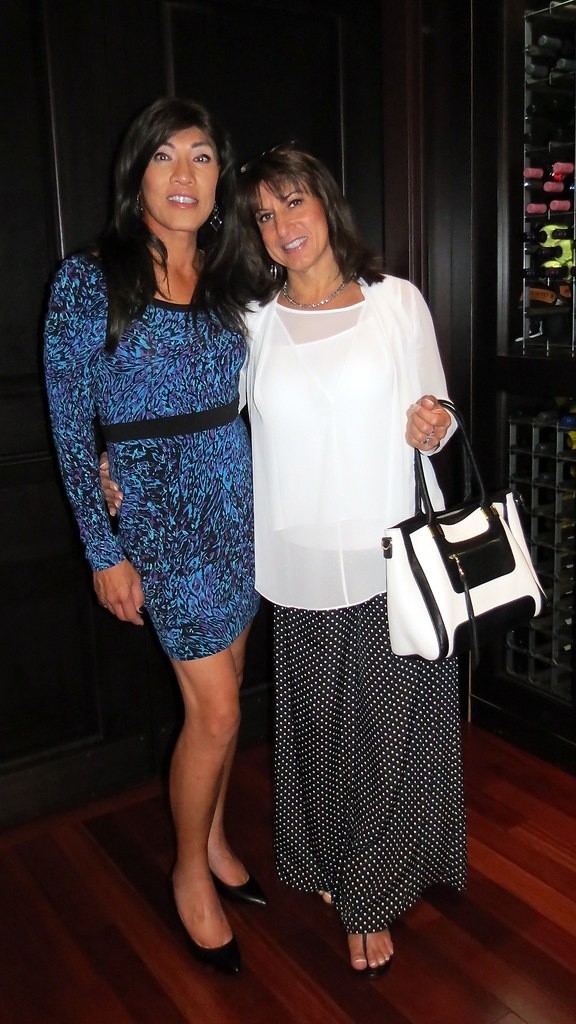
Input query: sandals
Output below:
<box><xmin>347</xmin><ymin>925</ymin><xmax>396</xmax><ymax>981</ymax></box>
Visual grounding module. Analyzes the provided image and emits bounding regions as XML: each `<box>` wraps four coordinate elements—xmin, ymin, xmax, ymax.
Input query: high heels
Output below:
<box><xmin>175</xmin><ymin>900</ymin><xmax>242</xmax><ymax>975</ymax></box>
<box><xmin>208</xmin><ymin>858</ymin><xmax>268</xmax><ymax>906</ymax></box>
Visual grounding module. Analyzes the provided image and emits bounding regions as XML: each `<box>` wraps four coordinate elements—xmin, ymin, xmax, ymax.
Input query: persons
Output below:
<box><xmin>44</xmin><ymin>99</ymin><xmax>267</xmax><ymax>975</ymax></box>
<box><xmin>96</xmin><ymin>147</ymin><xmax>471</xmax><ymax>983</ymax></box>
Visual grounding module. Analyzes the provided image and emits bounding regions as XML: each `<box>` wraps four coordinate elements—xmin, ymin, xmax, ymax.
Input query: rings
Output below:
<box><xmin>421</xmin><ymin>438</ymin><xmax>429</xmax><ymax>445</ymax></box>
<box><xmin>99</xmin><ymin>603</ymin><xmax>105</xmax><ymax>607</ymax></box>
<box><xmin>430</xmin><ymin>426</ymin><xmax>435</xmax><ymax>436</ymax></box>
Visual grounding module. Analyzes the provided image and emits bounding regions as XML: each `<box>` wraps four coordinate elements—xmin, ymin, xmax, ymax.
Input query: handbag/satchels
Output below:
<box><xmin>381</xmin><ymin>400</ymin><xmax>546</xmax><ymax>662</ymax></box>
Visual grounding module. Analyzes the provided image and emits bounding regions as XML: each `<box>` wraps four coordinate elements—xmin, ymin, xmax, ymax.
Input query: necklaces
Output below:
<box><xmin>283</xmin><ymin>277</ymin><xmax>344</xmax><ymax>308</ymax></box>
